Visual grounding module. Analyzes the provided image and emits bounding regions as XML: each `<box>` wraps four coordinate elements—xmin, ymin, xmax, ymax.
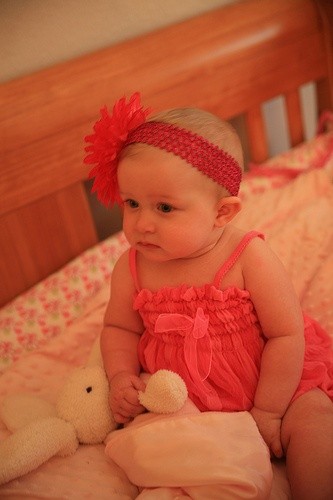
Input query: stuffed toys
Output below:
<box><xmin>0</xmin><ymin>333</ymin><xmax>187</xmax><ymax>486</ymax></box>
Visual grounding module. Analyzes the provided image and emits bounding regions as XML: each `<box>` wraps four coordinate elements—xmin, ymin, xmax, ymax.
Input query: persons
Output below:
<box><xmin>83</xmin><ymin>92</ymin><xmax>333</xmax><ymax>499</ymax></box>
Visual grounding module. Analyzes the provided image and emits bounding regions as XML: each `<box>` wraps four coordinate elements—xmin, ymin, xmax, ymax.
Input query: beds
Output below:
<box><xmin>0</xmin><ymin>125</ymin><xmax>333</xmax><ymax>500</ymax></box>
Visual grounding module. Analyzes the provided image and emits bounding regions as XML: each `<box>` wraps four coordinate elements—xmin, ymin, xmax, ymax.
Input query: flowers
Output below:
<box><xmin>84</xmin><ymin>92</ymin><xmax>156</xmax><ymax>210</ymax></box>
